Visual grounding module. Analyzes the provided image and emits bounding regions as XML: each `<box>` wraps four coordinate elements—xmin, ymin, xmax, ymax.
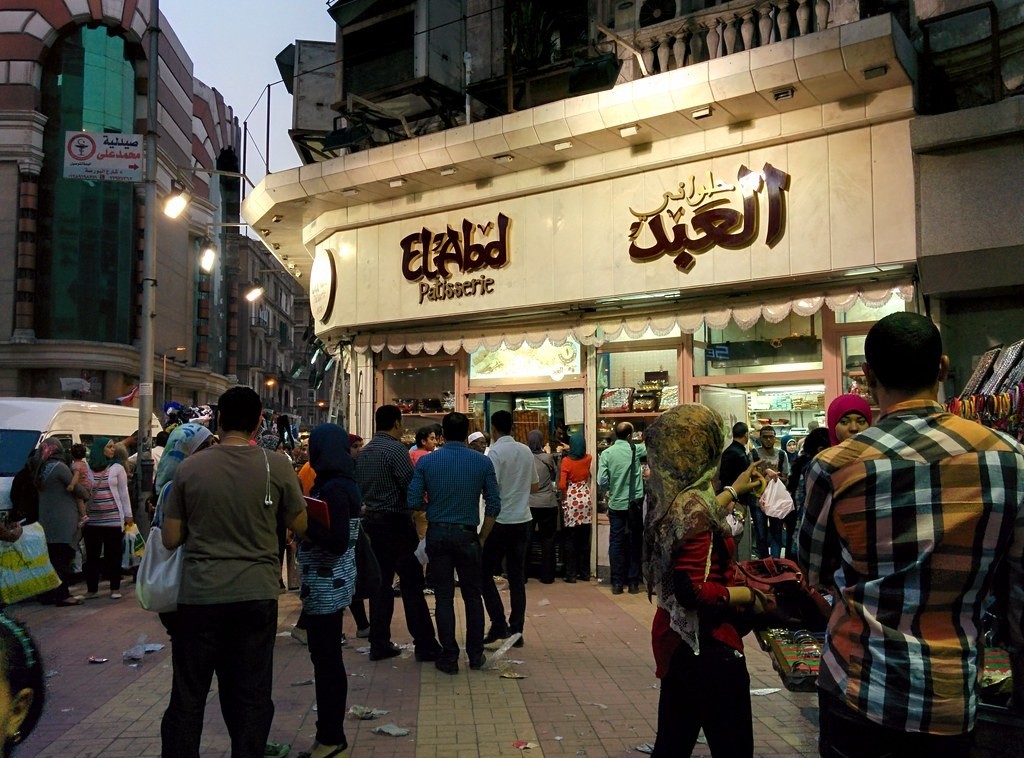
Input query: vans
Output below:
<box><xmin>0</xmin><ymin>395</ymin><xmax>164</xmax><ymax>577</ymax></box>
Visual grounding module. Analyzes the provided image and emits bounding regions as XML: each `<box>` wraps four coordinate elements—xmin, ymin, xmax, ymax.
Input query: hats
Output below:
<box><xmin>468</xmin><ymin>432</ymin><xmax>484</xmax><ymax>444</ymax></box>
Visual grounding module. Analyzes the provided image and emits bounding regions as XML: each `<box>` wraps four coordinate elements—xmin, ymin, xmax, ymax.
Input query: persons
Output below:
<box><xmin>796</xmin><ymin>311</ymin><xmax>1024</xmax><ymax>758</ymax></box>
<box><xmin>598</xmin><ymin>393</ymin><xmax>882</xmax><ymax>758</ymax></box>
<box><xmin>1</xmin><ymin>385</ymin><xmax>592</xmax><ymax>758</ymax></box>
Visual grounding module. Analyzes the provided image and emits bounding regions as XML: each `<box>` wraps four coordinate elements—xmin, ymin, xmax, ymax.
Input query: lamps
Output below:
<box><xmin>321</xmin><ymin>92</ymin><xmax>412</xmax><ymax>152</ymax></box>
<box><xmin>197</xmin><ymin>223</ymin><xmax>249</xmax><ymax>274</ymax></box>
<box><xmin>162</xmin><ymin>166</ymin><xmax>255</xmax><ymax>220</ymax></box>
<box><xmin>243</xmin><ymin>269</ymin><xmax>286</xmax><ymax>302</ymax></box>
<box><xmin>290</xmin><ymin>325</ymin><xmax>335</xmax><ymax>391</ymax></box>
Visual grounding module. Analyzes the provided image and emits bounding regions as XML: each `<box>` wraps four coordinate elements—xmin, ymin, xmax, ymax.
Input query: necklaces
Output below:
<box><xmin>220</xmin><ymin>435</ymin><xmax>251</xmax><ymax>443</ymax></box>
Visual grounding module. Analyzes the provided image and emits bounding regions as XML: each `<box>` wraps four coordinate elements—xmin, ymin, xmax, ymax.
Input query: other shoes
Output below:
<box><xmin>613</xmin><ymin>583</ymin><xmax>624</xmax><ymax>595</ymax></box>
<box><xmin>469</xmin><ymin>654</ymin><xmax>487</xmax><ymax>669</ymax></box>
<box><xmin>110</xmin><ymin>591</ymin><xmax>123</xmax><ymax>598</ymax></box>
<box><xmin>356</xmin><ymin>627</ymin><xmax>371</xmax><ymax>637</ymax></box>
<box><xmin>264</xmin><ymin>741</ymin><xmax>290</xmax><ymax>758</ymax></box>
<box><xmin>485</xmin><ymin>621</ymin><xmax>523</xmax><ymax>647</ymax></box>
<box><xmin>629</xmin><ymin>581</ymin><xmax>640</xmax><ymax>594</ymax></box>
<box><xmin>369</xmin><ymin>644</ymin><xmax>402</xmax><ymax>661</ymax></box>
<box><xmin>77</xmin><ymin>515</ymin><xmax>90</xmax><ymax>528</ymax></box>
<box><xmin>56</xmin><ymin>596</ymin><xmax>80</xmax><ymax>605</ymax></box>
<box><xmin>84</xmin><ymin>590</ymin><xmax>100</xmax><ymax>598</ymax></box>
<box><xmin>435</xmin><ymin>656</ymin><xmax>460</xmax><ymax>674</ymax></box>
<box><xmin>299</xmin><ymin>740</ymin><xmax>348</xmax><ymax>758</ymax></box>
<box><xmin>414</xmin><ymin>641</ymin><xmax>442</xmax><ymax>661</ymax></box>
<box><xmin>291</xmin><ymin>627</ymin><xmax>307</xmax><ymax>645</ymax></box>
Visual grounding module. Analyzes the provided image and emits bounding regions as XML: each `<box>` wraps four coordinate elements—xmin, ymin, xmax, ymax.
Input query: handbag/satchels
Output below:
<box><xmin>726</xmin><ymin>511</ymin><xmax>744</xmax><ymax>536</ymax></box>
<box><xmin>629</xmin><ymin>497</ymin><xmax>644</xmax><ymax>538</ymax></box>
<box><xmin>0</xmin><ymin>521</ymin><xmax>63</xmax><ymax>605</ymax></box>
<box><xmin>735</xmin><ymin>557</ymin><xmax>831</xmax><ymax>638</ymax></box>
<box><xmin>759</xmin><ymin>479</ymin><xmax>796</xmax><ymax>519</ymax></box>
<box><xmin>69</xmin><ymin>537</ymin><xmax>88</xmax><ymax>574</ymax></box>
<box><xmin>135</xmin><ymin>527</ymin><xmax>182</xmax><ymax>613</ymax></box>
<box><xmin>355</xmin><ymin>522</ymin><xmax>383</xmax><ymax>600</ymax></box>
<box><xmin>121</xmin><ymin>523</ymin><xmax>145</xmax><ymax>571</ymax></box>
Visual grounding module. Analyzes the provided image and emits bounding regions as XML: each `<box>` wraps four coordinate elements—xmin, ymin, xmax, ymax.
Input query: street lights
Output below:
<box><xmin>162</xmin><ymin>346</ymin><xmax>187</xmax><ymax>413</ymax></box>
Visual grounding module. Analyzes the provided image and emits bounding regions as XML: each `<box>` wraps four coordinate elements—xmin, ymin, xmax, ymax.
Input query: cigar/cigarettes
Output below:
<box><xmin>17</xmin><ymin>517</ymin><xmax>27</xmax><ymax>524</ymax></box>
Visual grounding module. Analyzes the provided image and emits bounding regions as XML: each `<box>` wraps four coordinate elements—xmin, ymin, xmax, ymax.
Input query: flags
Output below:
<box><xmin>116</xmin><ymin>386</ymin><xmax>139</xmax><ymax>403</ymax></box>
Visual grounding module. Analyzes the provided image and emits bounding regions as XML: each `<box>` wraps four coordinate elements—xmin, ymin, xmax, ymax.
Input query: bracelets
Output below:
<box><xmin>724</xmin><ymin>486</ymin><xmax>739</xmax><ymax>500</ymax></box>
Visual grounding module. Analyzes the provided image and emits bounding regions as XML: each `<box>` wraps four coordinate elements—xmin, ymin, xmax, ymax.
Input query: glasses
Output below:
<box><xmin>761</xmin><ymin>435</ymin><xmax>776</xmax><ymax>439</ymax></box>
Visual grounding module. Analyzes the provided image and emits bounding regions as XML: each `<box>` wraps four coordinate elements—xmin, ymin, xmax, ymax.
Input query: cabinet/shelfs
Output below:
<box><xmin>597</xmin><ymin>344</ymin><xmax>688</xmax><ymax>580</ymax></box>
<box><xmin>377</xmin><ymin>347</ymin><xmax>467</xmax><ymax>448</ymax></box>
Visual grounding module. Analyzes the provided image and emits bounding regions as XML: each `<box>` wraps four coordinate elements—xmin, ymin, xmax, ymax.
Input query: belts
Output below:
<box><xmin>432</xmin><ymin>521</ymin><xmax>477</xmax><ymax>534</ymax></box>
